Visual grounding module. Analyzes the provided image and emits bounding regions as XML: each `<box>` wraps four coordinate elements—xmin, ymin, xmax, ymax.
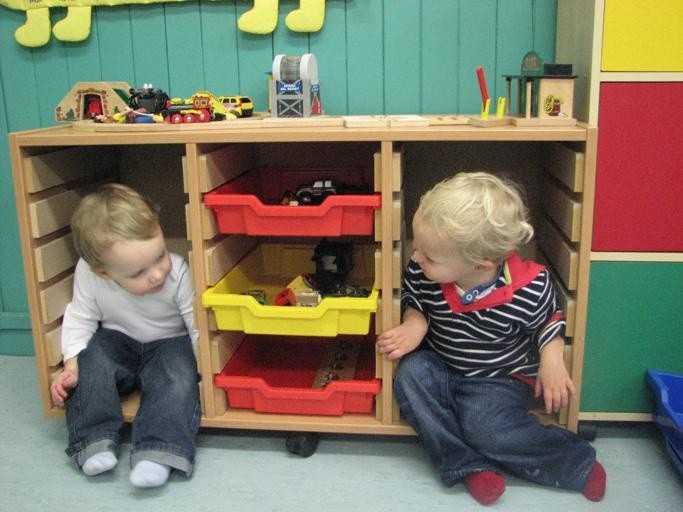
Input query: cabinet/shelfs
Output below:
<box><xmin>9</xmin><ymin>114</ymin><xmax>599</xmax><ymax>459</ymax></box>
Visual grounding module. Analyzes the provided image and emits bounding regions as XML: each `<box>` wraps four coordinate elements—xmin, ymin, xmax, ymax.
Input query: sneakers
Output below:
<box><xmin>285</xmin><ymin>432</ymin><xmax>318</xmax><ymax>457</ymax></box>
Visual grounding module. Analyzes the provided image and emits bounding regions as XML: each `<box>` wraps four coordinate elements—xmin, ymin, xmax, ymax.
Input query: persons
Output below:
<box><xmin>50</xmin><ymin>183</ymin><xmax>197</xmax><ymax>489</ymax></box>
<box><xmin>376</xmin><ymin>171</ymin><xmax>606</xmax><ymax>505</ymax></box>
